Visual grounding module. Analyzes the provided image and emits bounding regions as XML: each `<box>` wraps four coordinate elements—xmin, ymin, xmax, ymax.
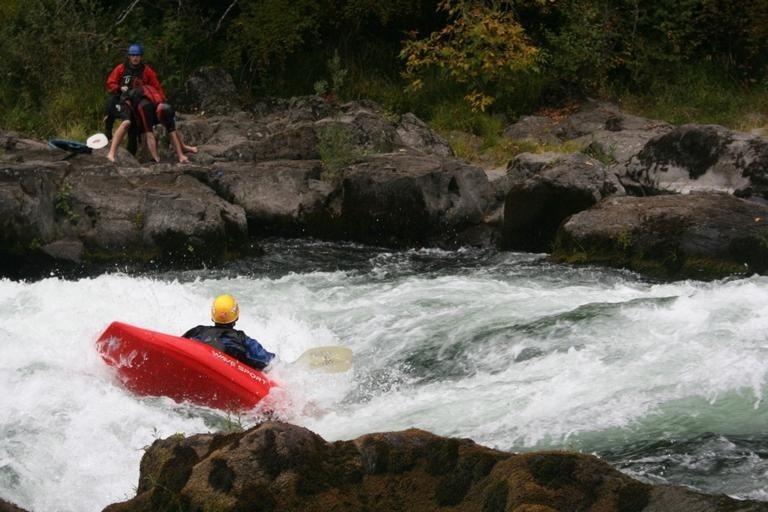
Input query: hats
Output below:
<box><xmin>86</xmin><ymin>133</ymin><xmax>108</xmax><ymax>150</ymax></box>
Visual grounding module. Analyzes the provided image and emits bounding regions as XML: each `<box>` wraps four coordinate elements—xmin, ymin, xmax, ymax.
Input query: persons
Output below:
<box><xmin>180</xmin><ymin>294</ymin><xmax>275</xmax><ymax>372</ymax></box>
<box><xmin>108</xmin><ymin>85</ymin><xmax>189</xmax><ymax>163</ymax></box>
<box><xmin>106</xmin><ymin>44</ymin><xmax>168</xmax><ymax>156</ymax></box>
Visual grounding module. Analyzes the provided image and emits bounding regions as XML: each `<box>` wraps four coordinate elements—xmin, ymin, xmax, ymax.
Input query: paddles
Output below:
<box><xmin>63</xmin><ymin>133</ymin><xmax>108</xmax><ymax>161</ymax></box>
<box><xmin>274</xmin><ymin>346</ymin><xmax>353</xmax><ymax>373</ymax></box>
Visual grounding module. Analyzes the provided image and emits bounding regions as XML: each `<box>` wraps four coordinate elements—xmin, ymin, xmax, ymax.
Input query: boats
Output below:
<box><xmin>95</xmin><ymin>321</ymin><xmax>323</xmax><ymax>421</ymax></box>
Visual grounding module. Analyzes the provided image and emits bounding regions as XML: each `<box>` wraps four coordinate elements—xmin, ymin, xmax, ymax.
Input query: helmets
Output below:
<box><xmin>127</xmin><ymin>45</ymin><xmax>142</xmax><ymax>55</ymax></box>
<box><xmin>211</xmin><ymin>295</ymin><xmax>239</xmax><ymax>324</ymax></box>
<box><xmin>156</xmin><ymin>102</ymin><xmax>175</xmax><ymax>122</ymax></box>
